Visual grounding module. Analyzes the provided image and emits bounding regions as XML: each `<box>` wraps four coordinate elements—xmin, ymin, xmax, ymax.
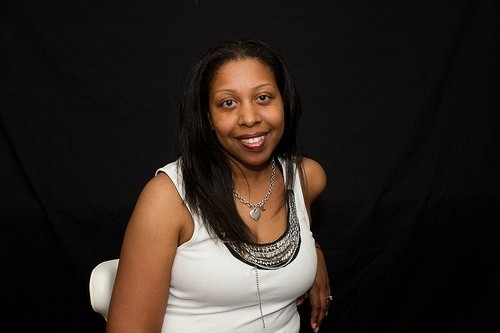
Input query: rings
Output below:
<box><xmin>326</xmin><ymin>295</ymin><xmax>333</xmax><ymax>301</ymax></box>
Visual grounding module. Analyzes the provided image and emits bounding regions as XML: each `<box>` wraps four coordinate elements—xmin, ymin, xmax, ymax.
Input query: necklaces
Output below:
<box><xmin>231</xmin><ymin>155</ymin><xmax>276</xmax><ymax>221</ymax></box>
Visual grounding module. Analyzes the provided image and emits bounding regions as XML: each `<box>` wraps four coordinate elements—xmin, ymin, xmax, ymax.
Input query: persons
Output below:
<box><xmin>104</xmin><ymin>41</ymin><xmax>336</xmax><ymax>333</ymax></box>
